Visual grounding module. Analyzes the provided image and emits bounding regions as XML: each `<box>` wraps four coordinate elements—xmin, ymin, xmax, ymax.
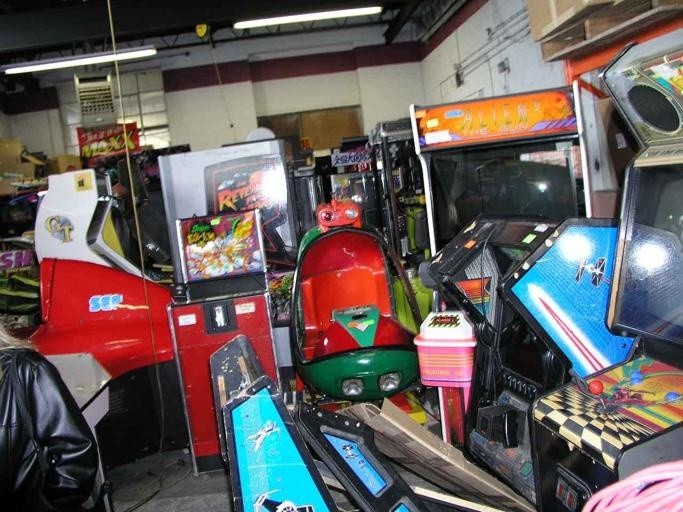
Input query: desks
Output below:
<box><xmin>45</xmin><ymin>352</ymin><xmax>112</xmax><ymax>512</ymax></box>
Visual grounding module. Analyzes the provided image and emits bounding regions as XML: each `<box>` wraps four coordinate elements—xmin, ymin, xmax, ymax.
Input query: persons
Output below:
<box><xmin>0</xmin><ymin>321</ymin><xmax>99</xmax><ymax>512</ymax></box>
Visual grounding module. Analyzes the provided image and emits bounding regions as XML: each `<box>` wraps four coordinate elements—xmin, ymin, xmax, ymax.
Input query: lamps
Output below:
<box><xmin>1</xmin><ymin>42</ymin><xmax>158</xmax><ymax>77</ymax></box>
<box><xmin>231</xmin><ymin>6</ymin><xmax>392</xmax><ymax>34</ymax></box>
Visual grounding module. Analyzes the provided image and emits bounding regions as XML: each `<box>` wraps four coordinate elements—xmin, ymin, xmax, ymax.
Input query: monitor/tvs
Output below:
<box><xmin>605</xmin><ymin>171</ymin><xmax>683</xmax><ymax>343</ymax></box>
<box><xmin>431</xmin><ymin>141</ymin><xmax>584</xmax><ymax>241</ymax></box>
